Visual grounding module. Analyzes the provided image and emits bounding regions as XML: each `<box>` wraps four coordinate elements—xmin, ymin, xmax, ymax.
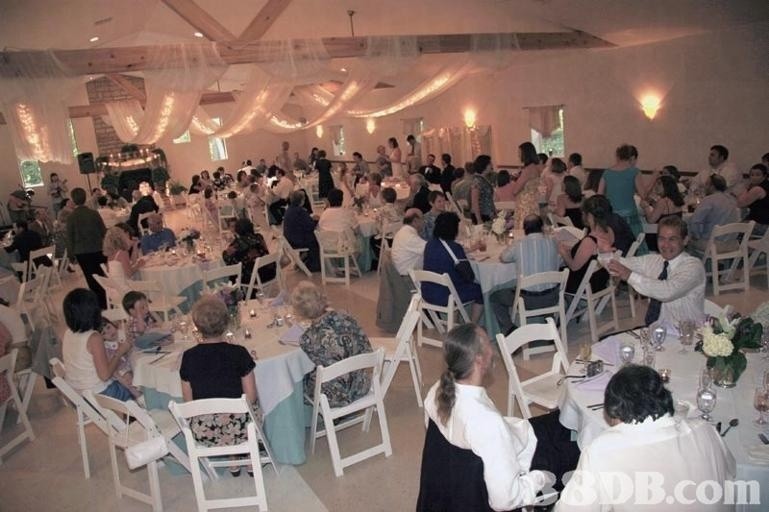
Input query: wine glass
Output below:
<box><xmin>579</xmin><ymin>344</ymin><xmax>592</xmax><ymax>378</ymax></box>
<box><xmin>652</xmin><ymin>323</ymin><xmax>666</xmax><ymax>352</ymax></box>
<box><xmin>146</xmin><ymin>238</ymin><xmax>225</xmax><ymax>261</ymax></box>
<box><xmin>639</xmin><ymin>327</ymin><xmax>655</xmax><ymax>369</ymax></box>
<box><xmin>618</xmin><ymin>343</ymin><xmax>635</xmax><ymax>365</ymax></box>
<box><xmin>753</xmin><ymin>386</ymin><xmax>768</xmax><ymax>427</ymax></box>
<box><xmin>679</xmin><ymin>331</ymin><xmax>690</xmax><ymax>354</ymax></box>
<box><xmin>698</xmin><ymin>388</ymin><xmax>716</xmax><ymax>424</ymax></box>
<box><xmin>162</xmin><ymin>313</ymin><xmax>193</xmax><ymax>341</ymax></box>
<box><xmin>256</xmin><ymin>291</ymin><xmax>265</xmax><ymax>311</ymax></box>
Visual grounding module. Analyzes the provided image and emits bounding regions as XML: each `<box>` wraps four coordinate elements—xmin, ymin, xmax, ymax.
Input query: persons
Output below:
<box><xmin>3</xmin><ymin>134</ymin><xmax>769</xmax><ymax>511</ymax></box>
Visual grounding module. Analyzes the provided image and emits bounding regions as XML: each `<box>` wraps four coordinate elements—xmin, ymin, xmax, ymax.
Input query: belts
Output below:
<box><xmin>520</xmin><ymin>285</ymin><xmax>560</xmax><ymax>295</ymax></box>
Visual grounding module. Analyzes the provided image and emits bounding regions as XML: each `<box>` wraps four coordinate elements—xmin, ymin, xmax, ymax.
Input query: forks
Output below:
<box><xmin>758</xmin><ymin>434</ymin><xmax>768</xmax><ymax>443</ymax></box>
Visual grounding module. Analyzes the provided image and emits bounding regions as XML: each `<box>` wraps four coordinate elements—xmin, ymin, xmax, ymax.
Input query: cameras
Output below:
<box><xmin>587</xmin><ymin>360</ymin><xmax>602</xmax><ymax>376</ymax></box>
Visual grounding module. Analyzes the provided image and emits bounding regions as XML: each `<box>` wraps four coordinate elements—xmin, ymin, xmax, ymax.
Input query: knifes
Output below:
<box><xmin>575</xmin><ymin>358</ymin><xmax>614</xmax><ymax>367</ymax></box>
<box><xmin>717</xmin><ymin>422</ymin><xmax>721</xmax><ymax>434</ymax></box>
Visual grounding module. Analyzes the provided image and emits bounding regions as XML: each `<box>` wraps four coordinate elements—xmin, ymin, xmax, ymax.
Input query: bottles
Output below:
<box><xmin>507</xmin><ymin>231</ymin><xmax>513</xmax><ymax>246</ymax></box>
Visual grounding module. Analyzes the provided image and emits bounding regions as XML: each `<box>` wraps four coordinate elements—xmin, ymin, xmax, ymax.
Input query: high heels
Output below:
<box><xmin>229</xmin><ymin>468</ymin><xmax>254</xmax><ymax>478</ymax></box>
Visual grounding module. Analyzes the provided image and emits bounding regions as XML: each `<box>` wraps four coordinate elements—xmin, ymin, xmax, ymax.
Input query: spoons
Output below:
<box><xmin>721</xmin><ymin>418</ymin><xmax>739</xmax><ymax>436</ymax></box>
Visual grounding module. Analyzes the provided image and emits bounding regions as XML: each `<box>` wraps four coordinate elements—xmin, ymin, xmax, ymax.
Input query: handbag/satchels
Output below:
<box><xmin>125</xmin><ymin>435</ymin><xmax>169</xmax><ymax>470</ymax></box>
<box><xmin>135</xmin><ymin>333</ymin><xmax>172</xmax><ymax>349</ymax></box>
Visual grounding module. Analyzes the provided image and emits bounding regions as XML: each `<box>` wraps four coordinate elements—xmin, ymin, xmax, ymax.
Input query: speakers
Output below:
<box><xmin>78</xmin><ymin>152</ymin><xmax>95</xmax><ymax>174</ymax></box>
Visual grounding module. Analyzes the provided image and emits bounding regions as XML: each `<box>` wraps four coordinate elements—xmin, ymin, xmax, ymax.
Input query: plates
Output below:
<box><xmin>576</xmin><ymin>375</ymin><xmax>612</xmax><ymax>391</ymax></box>
<box><xmin>136</xmin><ymin>333</ymin><xmax>163</xmax><ymax>349</ymax></box>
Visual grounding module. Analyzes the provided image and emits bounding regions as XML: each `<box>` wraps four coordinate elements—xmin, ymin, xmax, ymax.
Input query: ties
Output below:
<box><xmin>646</xmin><ymin>262</ymin><xmax>669</xmax><ymax>325</ymax></box>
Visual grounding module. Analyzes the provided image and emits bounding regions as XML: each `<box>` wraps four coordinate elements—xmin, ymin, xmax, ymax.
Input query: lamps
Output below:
<box><xmin>643</xmin><ymin>106</ymin><xmax>658</xmax><ymax>122</ymax></box>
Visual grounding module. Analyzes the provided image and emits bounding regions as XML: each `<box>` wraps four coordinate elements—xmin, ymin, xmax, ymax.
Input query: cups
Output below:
<box><xmin>478</xmin><ymin>237</ymin><xmax>487</xmax><ymax>251</ymax></box>
<box><xmin>658</xmin><ymin>369</ymin><xmax>670</xmax><ymax>382</ymax></box>
<box><xmin>700</xmin><ymin>366</ymin><xmax>713</xmax><ymax>389</ymax></box>
<box><xmin>680</xmin><ymin>319</ymin><xmax>695</xmax><ymax>346</ymax></box>
<box><xmin>244</xmin><ymin>307</ymin><xmax>292</xmax><ymax>338</ymax></box>
